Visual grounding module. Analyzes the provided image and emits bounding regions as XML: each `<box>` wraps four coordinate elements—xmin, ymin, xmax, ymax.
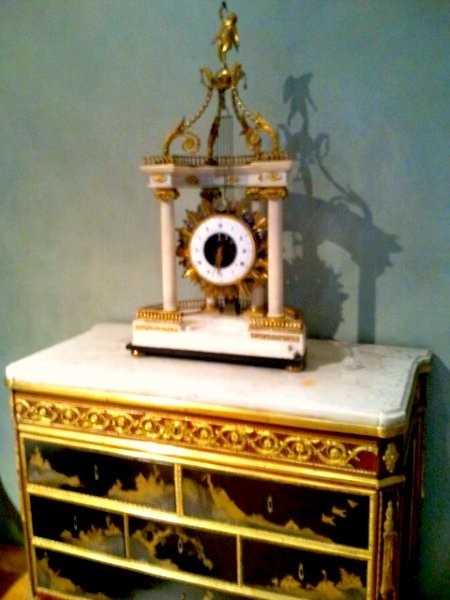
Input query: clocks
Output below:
<box><xmin>125</xmin><ymin>1</ymin><xmax>309</xmax><ymax>373</ymax></box>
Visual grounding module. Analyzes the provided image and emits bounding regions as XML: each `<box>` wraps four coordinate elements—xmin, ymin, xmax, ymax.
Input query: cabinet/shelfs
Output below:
<box><xmin>3</xmin><ymin>319</ymin><xmax>436</xmax><ymax>600</ymax></box>
